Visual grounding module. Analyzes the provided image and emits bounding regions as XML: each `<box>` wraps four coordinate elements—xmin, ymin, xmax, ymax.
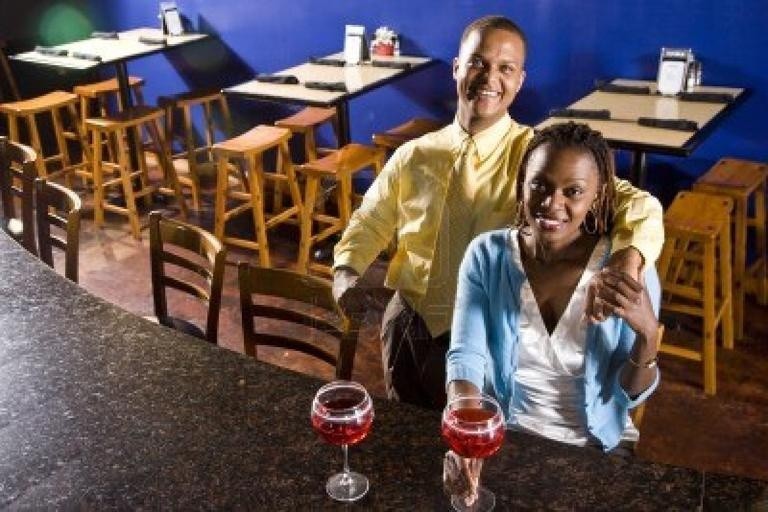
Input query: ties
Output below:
<box><xmin>423</xmin><ymin>138</ymin><xmax>474</xmax><ymax>339</ymax></box>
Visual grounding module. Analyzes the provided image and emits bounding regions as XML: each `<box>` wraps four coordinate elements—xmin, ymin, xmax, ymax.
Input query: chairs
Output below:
<box><xmin>138</xmin><ymin>210</ymin><xmax>226</xmax><ymax>346</ymax></box>
<box><xmin>597</xmin><ymin>321</ymin><xmax>664</xmax><ymax>458</ymax></box>
<box><xmin>239</xmin><ymin>262</ymin><xmax>361</xmax><ymax>391</ymax></box>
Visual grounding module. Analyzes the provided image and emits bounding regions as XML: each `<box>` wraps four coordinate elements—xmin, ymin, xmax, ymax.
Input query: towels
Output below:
<box><xmin>638</xmin><ymin>119</ymin><xmax>699</xmax><ymax>132</ymax></box>
<box><xmin>550</xmin><ymin>108</ymin><xmax>610</xmax><ymax>120</ymax></box>
<box><xmin>679</xmin><ymin>91</ymin><xmax>735</xmax><ymax>103</ymax></box>
<box><xmin>594</xmin><ymin>82</ymin><xmax>650</xmax><ymax>94</ymax></box>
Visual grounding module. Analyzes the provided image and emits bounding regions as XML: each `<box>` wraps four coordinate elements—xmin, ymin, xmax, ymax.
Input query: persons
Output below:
<box><xmin>330</xmin><ymin>14</ymin><xmax>664</xmax><ymax>413</ymax></box>
<box><xmin>441</xmin><ymin>120</ymin><xmax>661</xmax><ymax>501</ymax></box>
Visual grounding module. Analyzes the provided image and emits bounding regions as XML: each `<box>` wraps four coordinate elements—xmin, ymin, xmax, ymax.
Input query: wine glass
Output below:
<box><xmin>441</xmin><ymin>393</ymin><xmax>506</xmax><ymax>512</ymax></box>
<box><xmin>310</xmin><ymin>378</ymin><xmax>376</xmax><ymax>502</ymax></box>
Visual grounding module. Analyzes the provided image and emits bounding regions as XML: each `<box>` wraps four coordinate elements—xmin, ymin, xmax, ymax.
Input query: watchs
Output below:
<box><xmin>629</xmin><ymin>356</ymin><xmax>658</xmax><ymax>369</ymax></box>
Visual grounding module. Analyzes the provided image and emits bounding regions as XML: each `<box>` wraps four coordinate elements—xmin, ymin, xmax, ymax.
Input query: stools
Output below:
<box><xmin>654</xmin><ymin>191</ymin><xmax>735</xmax><ymax>395</ymax></box>
<box><xmin>683</xmin><ymin>159</ymin><xmax>768</xmax><ymax>340</ymax></box>
